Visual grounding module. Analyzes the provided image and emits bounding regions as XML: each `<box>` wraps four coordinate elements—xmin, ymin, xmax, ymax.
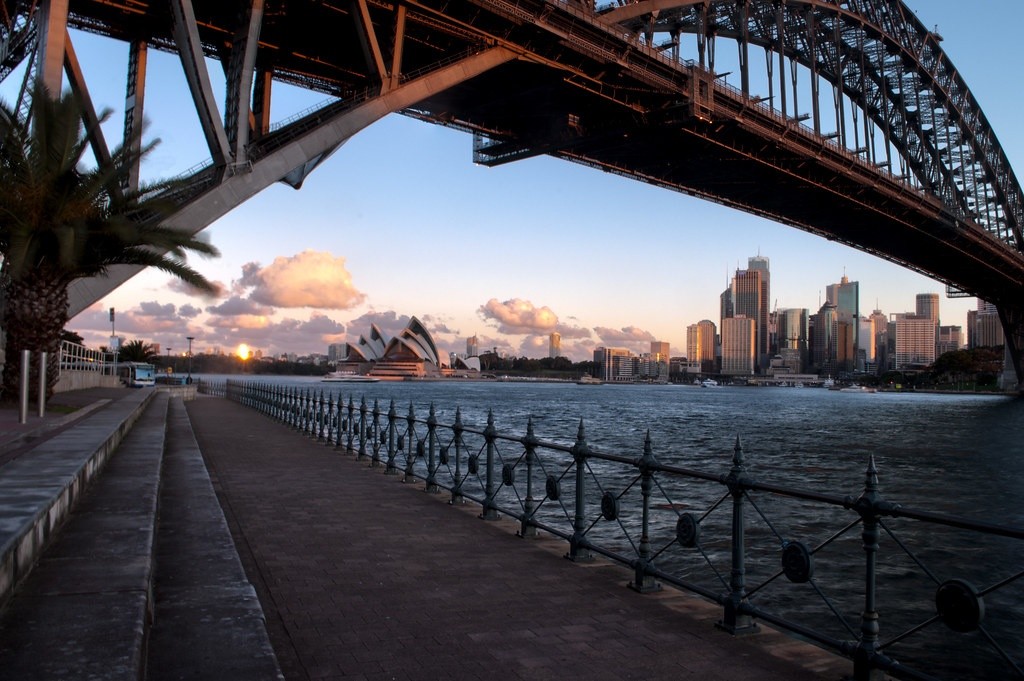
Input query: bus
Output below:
<box><xmin>104</xmin><ymin>361</ymin><xmax>157</xmax><ymax>388</ymax></box>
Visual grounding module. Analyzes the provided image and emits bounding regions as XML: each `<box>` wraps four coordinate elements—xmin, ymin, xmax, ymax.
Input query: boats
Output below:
<box><xmin>320</xmin><ymin>370</ymin><xmax>382</xmax><ymax>383</ymax></box>
<box><xmin>841</xmin><ymin>385</ymin><xmax>878</xmax><ymax>394</ymax></box>
<box><xmin>700</xmin><ymin>377</ymin><xmax>723</xmax><ymax>388</ymax></box>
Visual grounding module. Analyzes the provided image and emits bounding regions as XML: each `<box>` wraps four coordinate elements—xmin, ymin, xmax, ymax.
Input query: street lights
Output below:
<box><xmin>166</xmin><ymin>348</ymin><xmax>172</xmax><ymax>386</ymax></box>
<box><xmin>186</xmin><ymin>336</ymin><xmax>195</xmax><ymax>388</ymax></box>
